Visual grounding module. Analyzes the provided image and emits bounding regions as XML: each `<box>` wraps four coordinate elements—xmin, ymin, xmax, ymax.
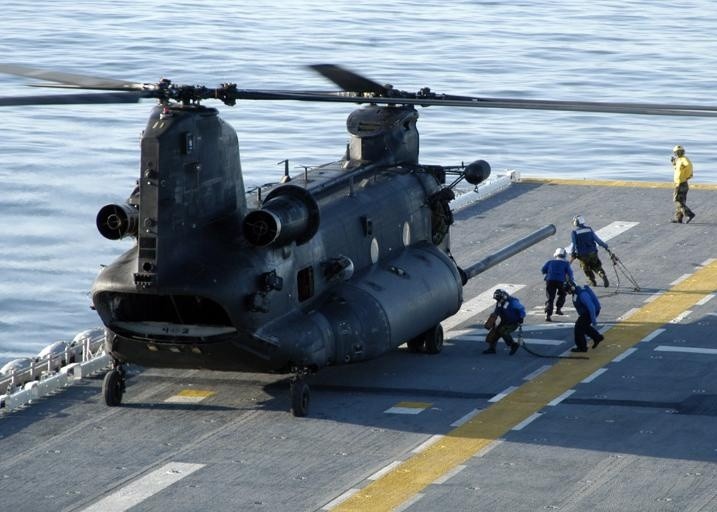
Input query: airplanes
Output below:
<box><xmin>0</xmin><ymin>62</ymin><xmax>712</xmax><ymax>419</ymax></box>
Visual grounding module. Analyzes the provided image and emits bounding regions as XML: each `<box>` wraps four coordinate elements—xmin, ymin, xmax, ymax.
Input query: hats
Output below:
<box><xmin>674</xmin><ymin>145</ymin><xmax>684</xmax><ymax>153</ymax></box>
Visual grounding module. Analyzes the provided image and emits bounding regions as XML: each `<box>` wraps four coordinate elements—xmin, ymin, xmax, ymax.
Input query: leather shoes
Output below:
<box><xmin>671</xmin><ymin>217</ymin><xmax>682</xmax><ymax>223</ymax></box>
<box><xmin>571</xmin><ymin>346</ymin><xmax>588</xmax><ymax>352</ymax></box>
<box><xmin>544</xmin><ymin>312</ymin><xmax>552</xmax><ymax>321</ymax></box>
<box><xmin>592</xmin><ymin>335</ymin><xmax>603</xmax><ymax>348</ymax></box>
<box><xmin>555</xmin><ymin>308</ymin><xmax>564</xmax><ymax>315</ymax></box>
<box><xmin>483</xmin><ymin>348</ymin><xmax>498</xmax><ymax>354</ymax></box>
<box><xmin>587</xmin><ymin>280</ymin><xmax>596</xmax><ymax>287</ymax></box>
<box><xmin>509</xmin><ymin>343</ymin><xmax>519</xmax><ymax>356</ymax></box>
<box><xmin>684</xmin><ymin>212</ymin><xmax>695</xmax><ymax>224</ymax></box>
<box><xmin>600</xmin><ymin>273</ymin><xmax>609</xmax><ymax>288</ymax></box>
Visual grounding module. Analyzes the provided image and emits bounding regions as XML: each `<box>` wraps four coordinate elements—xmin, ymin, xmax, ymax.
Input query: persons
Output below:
<box><xmin>668</xmin><ymin>145</ymin><xmax>696</xmax><ymax>224</ymax></box>
<box><xmin>567</xmin><ymin>215</ymin><xmax>609</xmax><ymax>288</ymax></box>
<box><xmin>540</xmin><ymin>248</ymin><xmax>574</xmax><ymax>321</ymax></box>
<box><xmin>562</xmin><ymin>280</ymin><xmax>605</xmax><ymax>353</ymax></box>
<box><xmin>481</xmin><ymin>288</ymin><xmax>527</xmax><ymax>355</ymax></box>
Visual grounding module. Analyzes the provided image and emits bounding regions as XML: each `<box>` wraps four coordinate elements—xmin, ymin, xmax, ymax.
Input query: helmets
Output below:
<box><xmin>562</xmin><ymin>280</ymin><xmax>576</xmax><ymax>295</ymax></box>
<box><xmin>493</xmin><ymin>289</ymin><xmax>508</xmax><ymax>303</ymax></box>
<box><xmin>572</xmin><ymin>214</ymin><xmax>585</xmax><ymax>226</ymax></box>
<box><xmin>553</xmin><ymin>247</ymin><xmax>568</xmax><ymax>258</ymax></box>
